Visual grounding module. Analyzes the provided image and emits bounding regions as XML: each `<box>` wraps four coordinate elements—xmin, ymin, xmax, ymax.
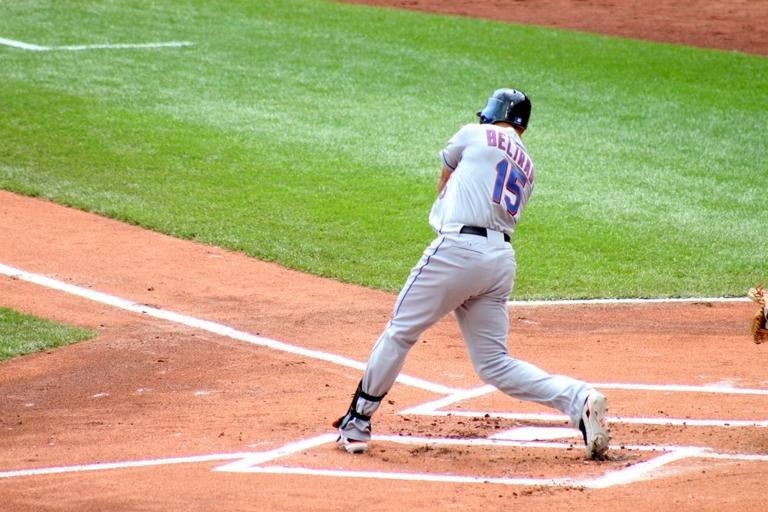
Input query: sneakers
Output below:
<box><xmin>579</xmin><ymin>388</ymin><xmax>613</xmax><ymax>461</ymax></box>
<box><xmin>340</xmin><ymin>433</ymin><xmax>368</xmax><ymax>454</ymax></box>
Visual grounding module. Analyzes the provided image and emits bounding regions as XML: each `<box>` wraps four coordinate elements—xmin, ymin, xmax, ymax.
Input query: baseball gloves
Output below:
<box><xmin>747</xmin><ymin>285</ymin><xmax>768</xmax><ymax>344</ymax></box>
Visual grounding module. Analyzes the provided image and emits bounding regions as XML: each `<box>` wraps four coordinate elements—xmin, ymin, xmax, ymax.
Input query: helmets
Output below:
<box><xmin>476</xmin><ymin>87</ymin><xmax>531</xmax><ymax>130</ymax></box>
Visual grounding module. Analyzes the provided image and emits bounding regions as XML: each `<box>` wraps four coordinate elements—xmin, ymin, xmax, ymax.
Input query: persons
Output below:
<box><xmin>333</xmin><ymin>86</ymin><xmax>611</xmax><ymax>460</ymax></box>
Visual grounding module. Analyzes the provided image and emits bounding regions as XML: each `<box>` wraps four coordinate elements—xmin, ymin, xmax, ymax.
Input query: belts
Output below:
<box><xmin>460</xmin><ymin>226</ymin><xmax>510</xmax><ymax>242</ymax></box>
